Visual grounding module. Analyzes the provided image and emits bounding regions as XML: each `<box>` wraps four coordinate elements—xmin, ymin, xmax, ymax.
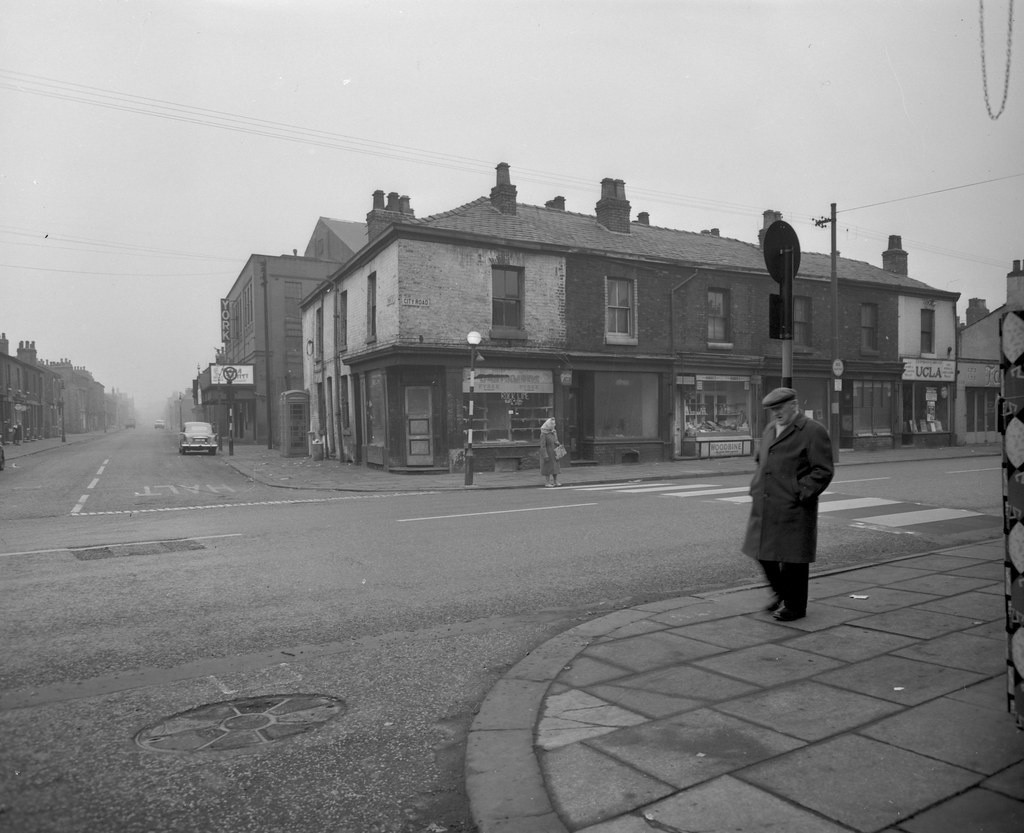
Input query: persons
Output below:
<box><xmin>12</xmin><ymin>420</ymin><xmax>23</xmax><ymax>446</ymax></box>
<box><xmin>540</xmin><ymin>417</ymin><xmax>562</xmax><ymax>488</ymax></box>
<box><xmin>739</xmin><ymin>387</ymin><xmax>834</xmax><ymax>620</ymax></box>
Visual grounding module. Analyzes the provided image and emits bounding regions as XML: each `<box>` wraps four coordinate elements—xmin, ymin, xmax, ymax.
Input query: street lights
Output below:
<box><xmin>178</xmin><ymin>395</ymin><xmax>183</xmax><ymax>432</ymax></box>
<box><xmin>465</xmin><ymin>330</ymin><xmax>481</xmax><ymax>486</ymax></box>
<box><xmin>60</xmin><ymin>385</ymin><xmax>66</xmax><ymax>442</ymax></box>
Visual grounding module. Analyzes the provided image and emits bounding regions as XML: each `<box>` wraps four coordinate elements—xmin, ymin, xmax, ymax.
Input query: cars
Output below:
<box><xmin>154</xmin><ymin>419</ymin><xmax>165</xmax><ymax>429</ymax></box>
<box><xmin>180</xmin><ymin>422</ymin><xmax>217</xmax><ymax>455</ymax></box>
<box><xmin>125</xmin><ymin>419</ymin><xmax>135</xmax><ymax>429</ymax></box>
<box><xmin>0</xmin><ymin>435</ymin><xmax>5</xmax><ymax>474</ymax></box>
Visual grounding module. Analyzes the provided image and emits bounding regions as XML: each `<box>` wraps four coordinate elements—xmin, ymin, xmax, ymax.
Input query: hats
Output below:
<box><xmin>761</xmin><ymin>388</ymin><xmax>796</xmax><ymax>409</ymax></box>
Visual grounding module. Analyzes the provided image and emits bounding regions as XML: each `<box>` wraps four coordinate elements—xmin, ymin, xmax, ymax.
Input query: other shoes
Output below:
<box><xmin>545</xmin><ymin>484</ymin><xmax>554</xmax><ymax>488</ymax></box>
<box><xmin>760</xmin><ymin>600</ymin><xmax>806</xmax><ymax>621</ymax></box>
<box><xmin>554</xmin><ymin>483</ymin><xmax>562</xmax><ymax>487</ymax></box>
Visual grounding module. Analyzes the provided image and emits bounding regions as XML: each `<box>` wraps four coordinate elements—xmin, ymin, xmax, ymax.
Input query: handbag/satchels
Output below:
<box><xmin>554</xmin><ymin>445</ymin><xmax>567</xmax><ymax>460</ymax></box>
